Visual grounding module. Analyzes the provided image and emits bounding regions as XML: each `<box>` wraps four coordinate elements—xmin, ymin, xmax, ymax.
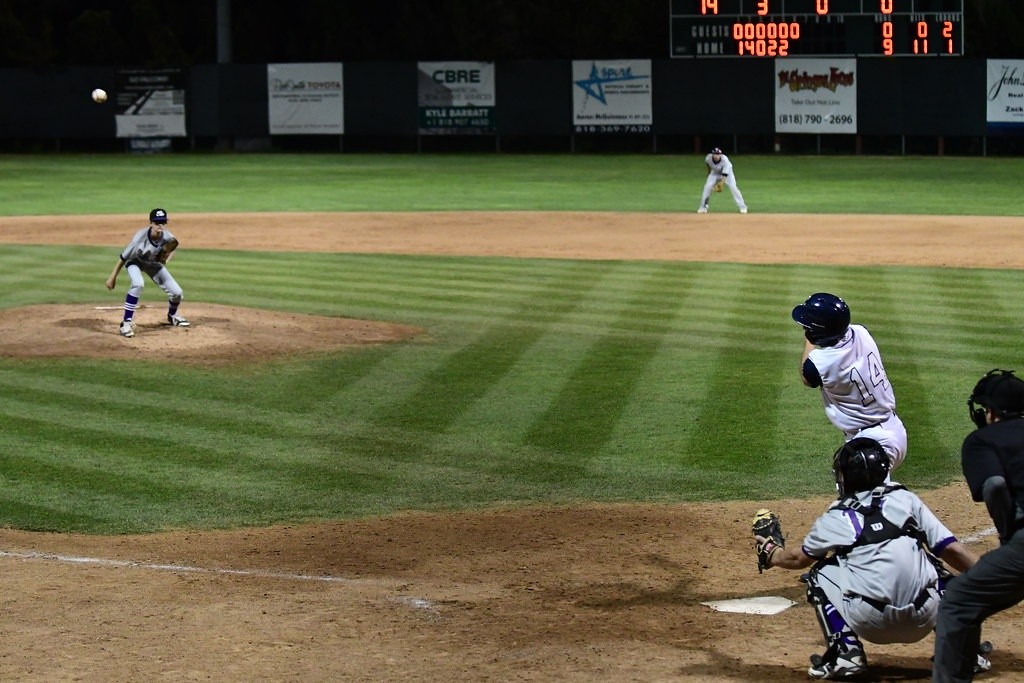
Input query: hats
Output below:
<box><xmin>150</xmin><ymin>209</ymin><xmax>169</xmax><ymax>223</ymax></box>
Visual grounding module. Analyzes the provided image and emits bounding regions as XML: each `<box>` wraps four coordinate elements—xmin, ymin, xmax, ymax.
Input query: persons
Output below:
<box><xmin>104</xmin><ymin>209</ymin><xmax>190</xmax><ymax>335</ymax></box>
<box><xmin>754</xmin><ymin>437</ymin><xmax>993</xmax><ymax>680</ymax></box>
<box><xmin>791</xmin><ymin>292</ymin><xmax>905</xmax><ymax>486</ymax></box>
<box><xmin>934</xmin><ymin>369</ymin><xmax>1024</xmax><ymax>682</ymax></box>
<box><xmin>696</xmin><ymin>147</ymin><xmax>747</xmax><ymax>215</ymax></box>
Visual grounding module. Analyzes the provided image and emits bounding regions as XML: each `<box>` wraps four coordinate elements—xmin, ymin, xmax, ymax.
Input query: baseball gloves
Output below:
<box><xmin>752</xmin><ymin>507</ymin><xmax>789</xmax><ymax>574</ymax></box>
<box><xmin>712</xmin><ymin>182</ymin><xmax>723</xmax><ymax>192</ymax></box>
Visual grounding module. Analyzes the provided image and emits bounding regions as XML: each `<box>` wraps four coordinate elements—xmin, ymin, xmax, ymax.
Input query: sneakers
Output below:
<box><xmin>168</xmin><ymin>315</ymin><xmax>190</xmax><ymax>326</ymax></box>
<box><xmin>696</xmin><ymin>208</ymin><xmax>709</xmax><ymax>213</ymax></box>
<box><xmin>808</xmin><ymin>651</ymin><xmax>870</xmax><ymax>677</ymax></box>
<box><xmin>740</xmin><ymin>208</ymin><xmax>748</xmax><ymax>213</ymax></box>
<box><xmin>972</xmin><ymin>656</ymin><xmax>993</xmax><ymax>674</ymax></box>
<box><xmin>119</xmin><ymin>324</ymin><xmax>135</xmax><ymax>337</ymax></box>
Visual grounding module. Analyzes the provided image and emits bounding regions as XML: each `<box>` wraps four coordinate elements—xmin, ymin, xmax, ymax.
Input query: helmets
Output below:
<box><xmin>792</xmin><ymin>292</ymin><xmax>850</xmax><ymax>346</ymax></box>
<box><xmin>966</xmin><ymin>370</ymin><xmax>1023</xmax><ymax>425</ymax></box>
<box><xmin>832</xmin><ymin>439</ymin><xmax>887</xmax><ymax>495</ymax></box>
<box><xmin>712</xmin><ymin>148</ymin><xmax>722</xmax><ymax>154</ymax></box>
